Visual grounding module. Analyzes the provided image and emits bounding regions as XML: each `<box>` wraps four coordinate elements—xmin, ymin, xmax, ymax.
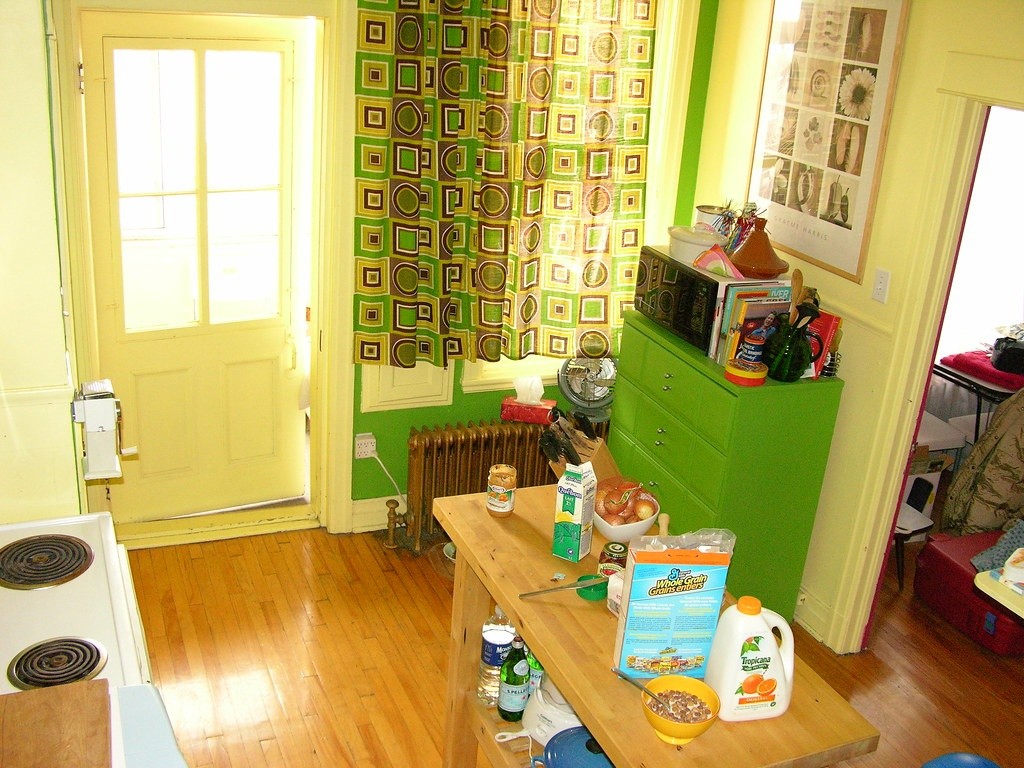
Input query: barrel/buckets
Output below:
<box><xmin>695</xmin><ymin>205</ymin><xmax>734</xmax><ymax>237</ymax></box>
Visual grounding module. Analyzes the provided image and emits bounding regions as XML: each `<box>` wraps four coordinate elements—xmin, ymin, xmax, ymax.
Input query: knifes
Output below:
<box><xmin>518</xmin><ymin>576</ymin><xmax>609</xmax><ymax>599</ymax></box>
<box><xmin>540</xmin><ymin>408</ymin><xmax>598</xmax><ymax>468</ymax></box>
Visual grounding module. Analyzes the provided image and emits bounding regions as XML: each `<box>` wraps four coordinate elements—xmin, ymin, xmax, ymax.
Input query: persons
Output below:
<box><xmin>751</xmin><ymin>310</ymin><xmax>778</xmax><ymax>339</ymax></box>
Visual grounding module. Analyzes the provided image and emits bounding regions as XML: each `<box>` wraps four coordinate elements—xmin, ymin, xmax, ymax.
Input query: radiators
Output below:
<box><xmin>383</xmin><ymin>420</ymin><xmax>610</xmax><ymax>549</ymax></box>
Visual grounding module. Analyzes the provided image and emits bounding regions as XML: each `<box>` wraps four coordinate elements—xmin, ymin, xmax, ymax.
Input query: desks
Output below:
<box><xmin>431</xmin><ymin>482</ymin><xmax>880</xmax><ymax>768</ymax></box>
<box><xmin>931</xmin><ymin>360</ymin><xmax>1015</xmax><ymax>444</ymax></box>
<box><xmin>974</xmin><ymin>567</ymin><xmax>1024</xmax><ymax>620</ymax></box>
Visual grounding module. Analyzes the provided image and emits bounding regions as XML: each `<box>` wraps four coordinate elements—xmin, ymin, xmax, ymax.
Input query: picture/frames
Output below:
<box><xmin>744</xmin><ymin>0</ymin><xmax>912</xmax><ymax>285</ymax></box>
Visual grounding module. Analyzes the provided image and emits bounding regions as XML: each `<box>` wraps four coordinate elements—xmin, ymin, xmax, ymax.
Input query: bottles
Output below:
<box><xmin>477</xmin><ymin>604</ymin><xmax>516</xmax><ymax>707</ymax></box>
<box><xmin>704</xmin><ymin>597</ymin><xmax>794</xmax><ymax>717</ymax></box>
<box><xmin>524</xmin><ymin>644</ymin><xmax>543</xmax><ymax>696</ymax></box>
<box><xmin>498</xmin><ymin>638</ymin><xmax>530</xmax><ymax>722</ymax></box>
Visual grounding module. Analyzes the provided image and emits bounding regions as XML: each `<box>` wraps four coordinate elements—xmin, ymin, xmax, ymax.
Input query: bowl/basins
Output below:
<box><xmin>594</xmin><ymin>494</ymin><xmax>660</xmax><ymax>545</ymax></box>
<box><xmin>640</xmin><ymin>675</ymin><xmax>721</xmax><ymax>744</ymax></box>
<box><xmin>667</xmin><ymin>223</ymin><xmax>728</xmax><ymax>263</ymax></box>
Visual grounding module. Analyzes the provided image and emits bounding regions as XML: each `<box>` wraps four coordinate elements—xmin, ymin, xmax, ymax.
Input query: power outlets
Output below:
<box><xmin>872</xmin><ymin>269</ymin><xmax>890</xmax><ymax>303</ymax></box>
<box><xmin>354</xmin><ymin>432</ymin><xmax>376</xmax><ymax>459</ymax></box>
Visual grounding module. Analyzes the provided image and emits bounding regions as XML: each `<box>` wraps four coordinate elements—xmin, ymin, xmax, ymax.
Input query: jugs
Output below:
<box><xmin>764</xmin><ymin>313</ymin><xmax>823</xmax><ymax>383</ymax></box>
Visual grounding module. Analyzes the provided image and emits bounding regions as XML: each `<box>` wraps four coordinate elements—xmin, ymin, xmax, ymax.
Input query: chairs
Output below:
<box><xmin>893</xmin><ymin>503</ymin><xmax>934</xmax><ymax>592</ymax></box>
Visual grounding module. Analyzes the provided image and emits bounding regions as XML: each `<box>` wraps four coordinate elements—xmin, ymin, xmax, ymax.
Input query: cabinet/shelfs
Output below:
<box><xmin>605</xmin><ymin>311</ymin><xmax>846</xmax><ymax>625</ymax></box>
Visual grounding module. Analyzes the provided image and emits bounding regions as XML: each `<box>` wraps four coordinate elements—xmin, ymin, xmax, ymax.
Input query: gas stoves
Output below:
<box><xmin>1</xmin><ymin>512</ymin><xmax>147</xmax><ymax>697</ymax></box>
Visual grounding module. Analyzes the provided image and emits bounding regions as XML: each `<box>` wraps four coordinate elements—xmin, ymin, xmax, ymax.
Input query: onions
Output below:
<box><xmin>594</xmin><ymin>481</ymin><xmax>655</xmax><ymax>526</ymax></box>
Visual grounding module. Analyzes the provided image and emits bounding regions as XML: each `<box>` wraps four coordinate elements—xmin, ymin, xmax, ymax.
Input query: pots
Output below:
<box><xmin>529</xmin><ymin>726</ymin><xmax>614</xmax><ymax>768</ymax></box>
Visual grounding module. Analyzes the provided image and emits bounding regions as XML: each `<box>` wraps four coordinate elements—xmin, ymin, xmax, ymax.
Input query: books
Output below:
<box><xmin>799</xmin><ymin>309</ymin><xmax>841</xmax><ymax>380</ymax></box>
<box><xmin>716</xmin><ymin>281</ymin><xmax>792</xmax><ymax>368</ymax></box>
<box><xmin>989</xmin><ymin>568</ymin><xmax>1024</xmax><ymax>594</ymax></box>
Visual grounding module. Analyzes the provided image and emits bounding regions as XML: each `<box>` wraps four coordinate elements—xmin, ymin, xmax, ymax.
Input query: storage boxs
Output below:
<box><xmin>912</xmin><ymin>530</ymin><xmax>1024</xmax><ymax>655</ymax></box>
<box><xmin>612</xmin><ymin>531</ymin><xmax>731</xmax><ymax>678</ymax></box>
<box><xmin>912</xmin><ymin>410</ymin><xmax>1001</xmax><ymax>473</ymax></box>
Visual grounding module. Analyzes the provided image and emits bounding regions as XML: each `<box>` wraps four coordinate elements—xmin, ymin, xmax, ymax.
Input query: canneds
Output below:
<box><xmin>485</xmin><ymin>464</ymin><xmax>517</xmax><ymax>519</ymax></box>
<box><xmin>596</xmin><ymin>542</ymin><xmax>628</xmax><ymax>579</ymax></box>
<box><xmin>695</xmin><ymin>205</ymin><xmax>726</xmax><ymax>233</ymax></box>
<box><xmin>741</xmin><ymin>334</ymin><xmax>765</xmax><ymax>364</ymax></box>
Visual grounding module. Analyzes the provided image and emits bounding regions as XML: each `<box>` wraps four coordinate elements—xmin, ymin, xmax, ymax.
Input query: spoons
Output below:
<box><xmin>611</xmin><ymin>668</ymin><xmax>680</xmax><ymax>719</ymax></box>
